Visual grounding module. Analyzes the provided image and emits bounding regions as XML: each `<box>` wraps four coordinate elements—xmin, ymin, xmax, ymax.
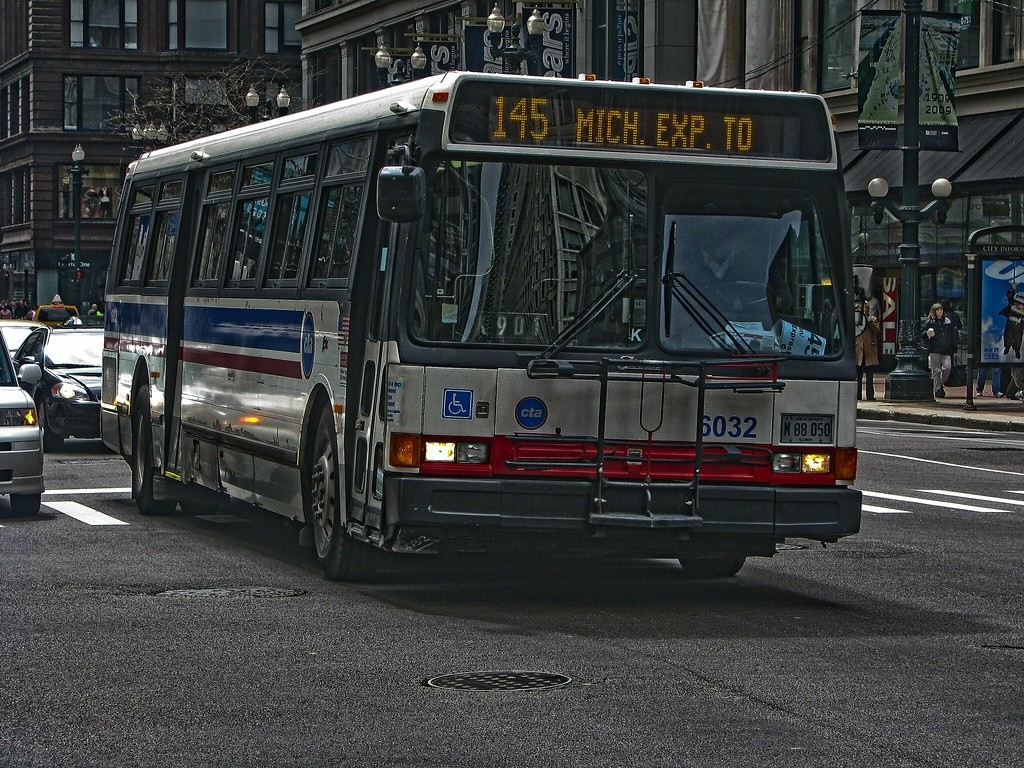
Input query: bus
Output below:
<box><xmin>95</xmin><ymin>72</ymin><xmax>864</xmax><ymax>589</ymax></box>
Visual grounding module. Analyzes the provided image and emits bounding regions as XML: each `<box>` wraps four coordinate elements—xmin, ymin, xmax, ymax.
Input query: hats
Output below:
<box><xmin>1013</xmin><ymin>292</ymin><xmax>1024</xmax><ymax>304</ymax></box>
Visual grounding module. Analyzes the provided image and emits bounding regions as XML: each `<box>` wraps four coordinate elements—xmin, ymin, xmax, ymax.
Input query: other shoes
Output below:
<box><xmin>935</xmin><ymin>385</ymin><xmax>946</xmax><ymax>398</ymax></box>
<box><xmin>976</xmin><ymin>392</ymin><xmax>982</xmax><ymax>396</ymax></box>
<box><xmin>993</xmin><ymin>392</ymin><xmax>1003</xmax><ymax>398</ymax></box>
<box><xmin>1004</xmin><ymin>347</ymin><xmax>1009</xmax><ymax>355</ymax></box>
<box><xmin>1015</xmin><ymin>352</ymin><xmax>1020</xmax><ymax>359</ymax></box>
<box><xmin>867</xmin><ymin>397</ymin><xmax>877</xmax><ymax>400</ymax></box>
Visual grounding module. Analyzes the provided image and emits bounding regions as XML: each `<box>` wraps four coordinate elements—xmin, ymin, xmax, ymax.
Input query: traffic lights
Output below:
<box><xmin>75</xmin><ymin>269</ymin><xmax>83</xmax><ymax>290</ymax></box>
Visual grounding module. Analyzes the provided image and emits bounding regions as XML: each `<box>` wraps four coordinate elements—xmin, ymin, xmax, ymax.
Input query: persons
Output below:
<box><xmin>0</xmin><ymin>298</ymin><xmax>39</xmax><ymax>321</ymax></box>
<box><xmin>920</xmin><ymin>303</ymin><xmax>959</xmax><ymax>398</ymax></box>
<box><xmin>998</xmin><ymin>282</ymin><xmax>1024</xmax><ymax>359</ymax></box>
<box><xmin>854</xmin><ymin>291</ymin><xmax>881</xmax><ymax>401</ymax></box>
<box><xmin>976</xmin><ymin>366</ymin><xmax>1024</xmax><ymax>404</ymax></box>
<box><xmin>52</xmin><ymin>294</ymin><xmax>62</xmax><ymax>305</ymax></box>
<box><xmin>679</xmin><ymin>221</ymin><xmax>748</xmax><ymax>329</ymax></box>
<box><xmin>88</xmin><ymin>304</ymin><xmax>102</xmax><ymax>316</ymax></box>
<box><xmin>852</xmin><ymin>274</ymin><xmax>865</xmax><ymax>302</ymax></box>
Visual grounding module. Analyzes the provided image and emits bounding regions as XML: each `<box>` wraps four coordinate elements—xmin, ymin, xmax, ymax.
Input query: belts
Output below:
<box><xmin>1011</xmin><ymin>321</ymin><xmax>1020</xmax><ymax>326</ymax></box>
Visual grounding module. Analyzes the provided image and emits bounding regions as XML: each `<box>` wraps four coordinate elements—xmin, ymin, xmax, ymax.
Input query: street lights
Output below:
<box><xmin>855</xmin><ymin>135</ymin><xmax>962</xmax><ymax>404</ymax></box>
<box><xmin>24</xmin><ymin>257</ymin><xmax>30</xmax><ymax>305</ymax></box>
<box><xmin>69</xmin><ymin>144</ymin><xmax>86</xmax><ymax>313</ymax></box>
<box><xmin>2</xmin><ymin>262</ymin><xmax>14</xmax><ymax>301</ymax></box>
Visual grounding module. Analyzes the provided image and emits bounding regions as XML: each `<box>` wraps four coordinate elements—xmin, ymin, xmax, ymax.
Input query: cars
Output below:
<box><xmin>64</xmin><ymin>314</ymin><xmax>107</xmax><ymax>329</ymax></box>
<box><xmin>0</xmin><ymin>320</ymin><xmax>46</xmax><ymax>362</ymax></box>
<box><xmin>0</xmin><ymin>329</ymin><xmax>44</xmax><ymax>517</ymax></box>
<box><xmin>12</xmin><ymin>328</ymin><xmax>108</xmax><ymax>444</ymax></box>
<box><xmin>32</xmin><ymin>304</ymin><xmax>80</xmax><ymax>330</ymax></box>
